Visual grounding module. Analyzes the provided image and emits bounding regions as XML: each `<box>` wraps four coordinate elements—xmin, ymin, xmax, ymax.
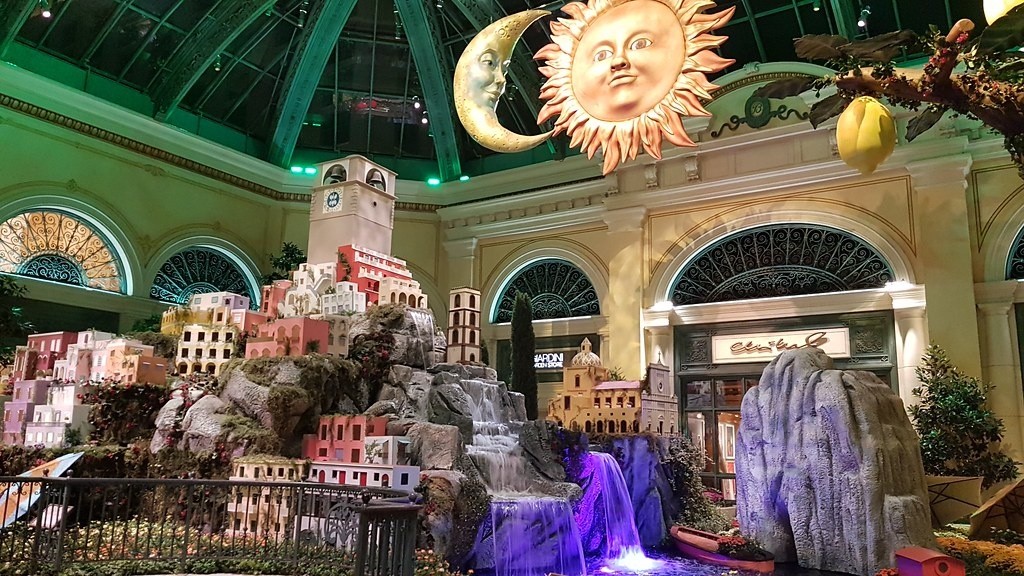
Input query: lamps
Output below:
<box><xmin>813</xmin><ymin>0</ymin><xmax>820</xmax><ymax>11</ymax></box>
<box><xmin>213</xmin><ymin>56</ymin><xmax>220</xmax><ymax>71</ymax></box>
<box><xmin>413</xmin><ymin>98</ymin><xmax>420</xmax><ymax>109</ymax></box>
<box><xmin>297</xmin><ymin>12</ymin><xmax>303</xmax><ymax>26</ymax></box>
<box><xmin>395</xmin><ymin>28</ymin><xmax>400</xmax><ymax>39</ymax></box>
<box><xmin>393</xmin><ymin>4</ymin><xmax>398</xmax><ymax>13</ymax></box>
<box><xmin>508</xmin><ymin>85</ymin><xmax>517</xmax><ymax>100</ymax></box>
<box><xmin>299</xmin><ymin>0</ymin><xmax>307</xmax><ymax>13</ymax></box>
<box><xmin>857</xmin><ymin>9</ymin><xmax>871</xmax><ymax>27</ymax></box>
<box><xmin>41</xmin><ymin>0</ymin><xmax>50</xmax><ymax>17</ymax></box>
<box><xmin>436</xmin><ymin>0</ymin><xmax>443</xmax><ymax>7</ymax></box>
<box><xmin>395</xmin><ymin>16</ymin><xmax>402</xmax><ymax>27</ymax></box>
<box><xmin>422</xmin><ymin>113</ymin><xmax>427</xmax><ymax>124</ymax></box>
